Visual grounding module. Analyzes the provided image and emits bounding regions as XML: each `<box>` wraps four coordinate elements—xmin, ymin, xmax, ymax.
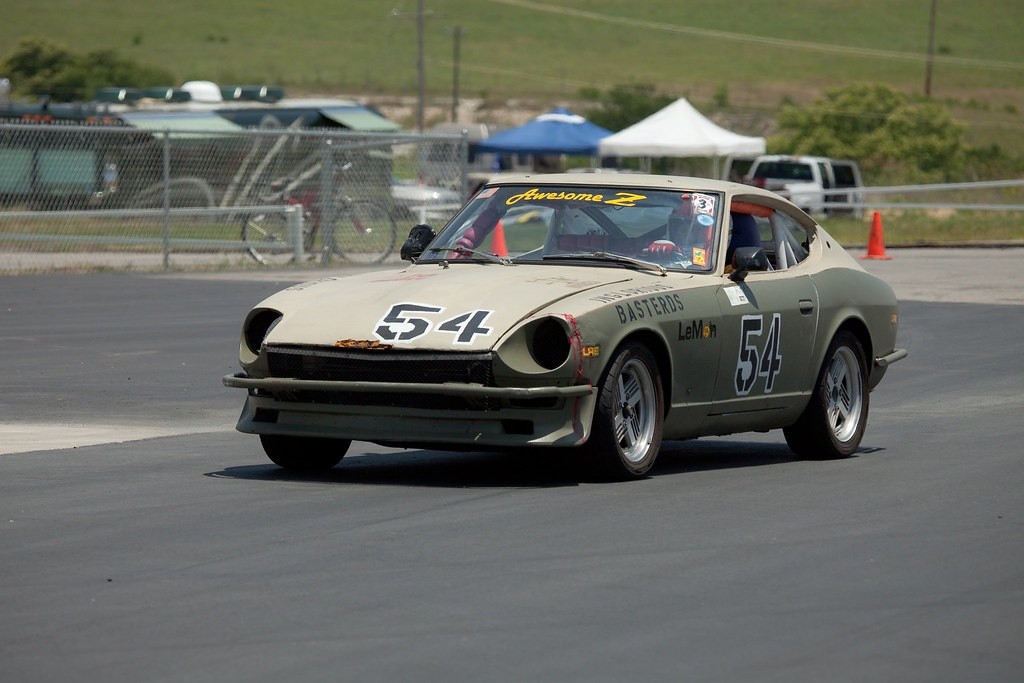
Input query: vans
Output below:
<box><xmin>722</xmin><ymin>152</ymin><xmax>866</xmax><ymax>225</ymax></box>
<box><xmin>415</xmin><ymin>121</ymin><xmax>562</xmax><ymax>191</ymax></box>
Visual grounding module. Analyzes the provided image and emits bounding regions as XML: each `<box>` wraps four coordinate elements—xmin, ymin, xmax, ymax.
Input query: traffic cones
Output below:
<box><xmin>490</xmin><ymin>218</ymin><xmax>508</xmax><ymax>257</ymax></box>
<box><xmin>859</xmin><ymin>212</ymin><xmax>895</xmax><ymax>261</ymax></box>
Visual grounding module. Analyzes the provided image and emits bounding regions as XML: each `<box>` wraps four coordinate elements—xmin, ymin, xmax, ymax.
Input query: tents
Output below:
<box><xmin>473</xmin><ymin>103</ymin><xmax>614</xmax><ymax>174</ymax></box>
<box><xmin>593</xmin><ymin>95</ymin><xmax>769</xmax><ymax>184</ymax></box>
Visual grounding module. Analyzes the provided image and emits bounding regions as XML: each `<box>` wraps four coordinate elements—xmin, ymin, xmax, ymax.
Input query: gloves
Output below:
<box><xmin>649</xmin><ymin>237</ymin><xmax>685</xmax><ymax>257</ymax></box>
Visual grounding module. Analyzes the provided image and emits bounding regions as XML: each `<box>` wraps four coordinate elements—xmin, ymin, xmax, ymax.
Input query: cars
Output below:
<box><xmin>388</xmin><ymin>170</ymin><xmax>495</xmax><ymax>223</ymax></box>
<box><xmin>220</xmin><ymin>171</ymin><xmax>909</xmax><ymax>484</ymax></box>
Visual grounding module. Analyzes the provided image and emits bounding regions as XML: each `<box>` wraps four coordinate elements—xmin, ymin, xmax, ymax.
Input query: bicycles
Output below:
<box><xmin>240</xmin><ymin>156</ymin><xmax>397</xmax><ymax>270</ymax></box>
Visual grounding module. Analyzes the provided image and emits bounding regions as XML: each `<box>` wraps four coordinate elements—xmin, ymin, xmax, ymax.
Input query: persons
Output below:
<box><xmin>640</xmin><ymin>193</ymin><xmax>736</xmax><ymax>277</ymax></box>
